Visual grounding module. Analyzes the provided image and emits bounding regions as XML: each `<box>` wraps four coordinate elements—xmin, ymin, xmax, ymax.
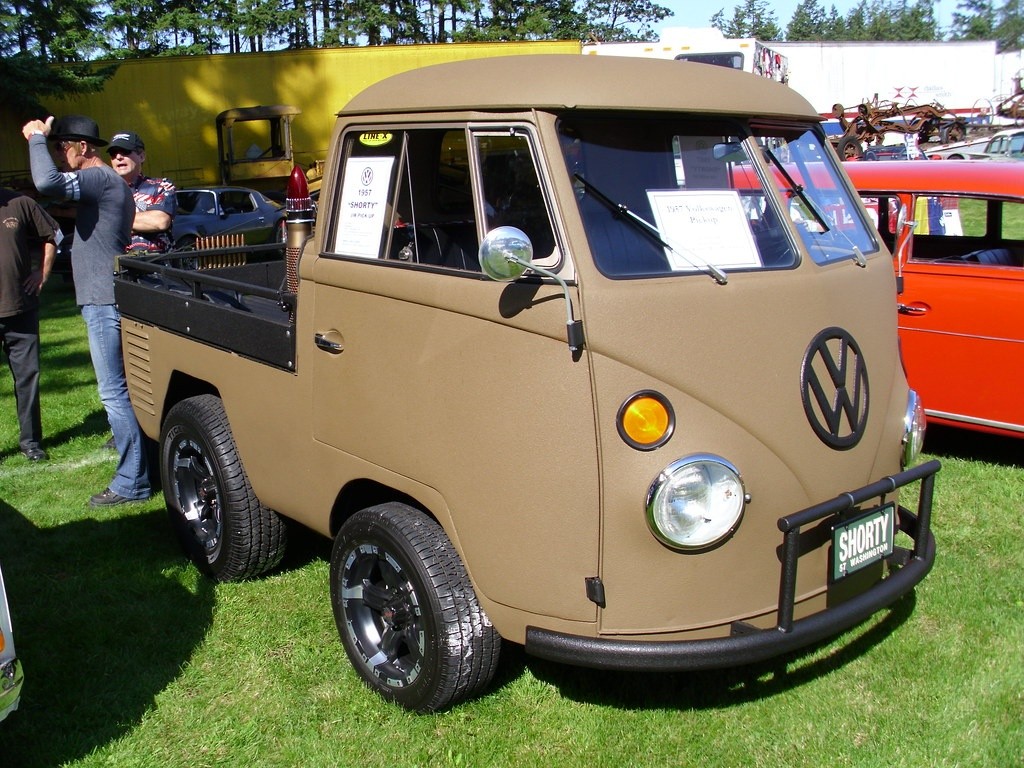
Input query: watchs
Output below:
<box><xmin>27</xmin><ymin>130</ymin><xmax>45</xmax><ymax>142</ymax></box>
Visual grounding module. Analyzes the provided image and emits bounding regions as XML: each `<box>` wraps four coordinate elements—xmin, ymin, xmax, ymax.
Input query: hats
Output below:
<box><xmin>47</xmin><ymin>114</ymin><xmax>109</xmax><ymax>147</ymax></box>
<box><xmin>106</xmin><ymin>131</ymin><xmax>145</xmax><ymax>151</ymax></box>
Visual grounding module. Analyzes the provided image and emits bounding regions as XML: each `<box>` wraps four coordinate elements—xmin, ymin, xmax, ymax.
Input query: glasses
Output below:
<box><xmin>54</xmin><ymin>143</ymin><xmax>72</xmax><ymax>153</ymax></box>
<box><xmin>109</xmin><ymin>147</ymin><xmax>132</xmax><ymax>157</ymax></box>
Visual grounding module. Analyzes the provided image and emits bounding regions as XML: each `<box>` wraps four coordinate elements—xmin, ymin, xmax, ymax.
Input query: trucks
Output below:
<box><xmin>113</xmin><ymin>55</ymin><xmax>942</xmax><ymax>714</ymax></box>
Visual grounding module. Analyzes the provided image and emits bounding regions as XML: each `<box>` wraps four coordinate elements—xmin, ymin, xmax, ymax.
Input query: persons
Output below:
<box><xmin>105</xmin><ymin>131</ymin><xmax>178</xmax><ymax>258</ymax></box>
<box><xmin>21</xmin><ymin>116</ymin><xmax>162</xmax><ymax>508</ymax></box>
<box><xmin>0</xmin><ymin>182</ymin><xmax>65</xmax><ymax>462</ymax></box>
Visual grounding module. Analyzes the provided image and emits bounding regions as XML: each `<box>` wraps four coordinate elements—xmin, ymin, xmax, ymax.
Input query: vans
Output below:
<box><xmin>728</xmin><ymin>160</ymin><xmax>1024</xmax><ymax>439</ymax></box>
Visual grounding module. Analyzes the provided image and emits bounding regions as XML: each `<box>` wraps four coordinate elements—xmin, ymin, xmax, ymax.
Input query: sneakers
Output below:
<box><xmin>21</xmin><ymin>448</ymin><xmax>48</xmax><ymax>462</ymax></box>
<box><xmin>101</xmin><ymin>435</ymin><xmax>117</xmax><ymax>451</ymax></box>
<box><xmin>90</xmin><ymin>487</ymin><xmax>133</xmax><ymax>507</ymax></box>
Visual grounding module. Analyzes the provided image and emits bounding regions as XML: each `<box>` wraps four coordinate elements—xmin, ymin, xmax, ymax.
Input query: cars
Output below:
<box><xmin>49</xmin><ymin>186</ymin><xmax>287</xmax><ymax>272</ymax></box>
